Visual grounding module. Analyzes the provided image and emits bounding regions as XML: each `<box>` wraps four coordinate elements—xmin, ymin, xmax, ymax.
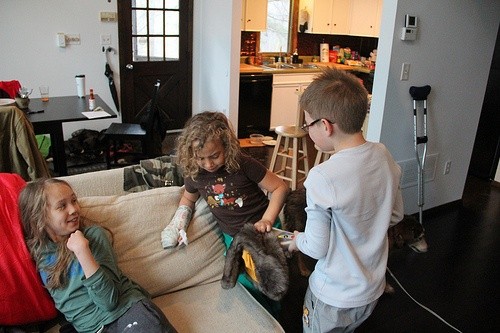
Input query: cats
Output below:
<box><xmin>283</xmin><ymin>187</ymin><xmax>317</xmax><ymax>272</ymax></box>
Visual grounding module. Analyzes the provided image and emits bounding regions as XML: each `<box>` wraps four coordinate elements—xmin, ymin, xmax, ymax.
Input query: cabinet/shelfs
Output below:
<box><xmin>304</xmin><ymin>0</ymin><xmax>383</xmax><ymax>37</ymax></box>
<box><xmin>238</xmin><ymin>71</ymin><xmax>376</xmax><ymax>141</ymax></box>
<box><xmin>241</xmin><ymin>0</ymin><xmax>268</xmax><ymax>31</ymax></box>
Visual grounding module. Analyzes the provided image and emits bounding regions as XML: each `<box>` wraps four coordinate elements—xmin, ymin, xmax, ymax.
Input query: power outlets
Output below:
<box><xmin>444</xmin><ymin>160</ymin><xmax>452</xmax><ymax>174</ymax></box>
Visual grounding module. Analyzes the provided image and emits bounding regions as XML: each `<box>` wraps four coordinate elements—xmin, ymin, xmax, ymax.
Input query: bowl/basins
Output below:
<box><xmin>249</xmin><ymin>133</ymin><xmax>264</xmax><ymax>145</ymax></box>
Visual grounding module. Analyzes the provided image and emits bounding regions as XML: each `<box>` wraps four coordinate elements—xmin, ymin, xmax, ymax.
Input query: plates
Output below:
<box><xmin>262</xmin><ymin>140</ymin><xmax>277</xmax><ymax>145</ymax></box>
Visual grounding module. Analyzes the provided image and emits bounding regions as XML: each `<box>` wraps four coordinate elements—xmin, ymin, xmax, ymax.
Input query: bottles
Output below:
<box><xmin>293</xmin><ymin>48</ymin><xmax>299</xmax><ymax>64</ymax></box>
<box><xmin>89</xmin><ymin>88</ymin><xmax>96</xmax><ymax>111</ymax></box>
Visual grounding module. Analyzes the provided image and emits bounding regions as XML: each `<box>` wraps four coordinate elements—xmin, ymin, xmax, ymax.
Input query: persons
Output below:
<box><xmin>16</xmin><ymin>179</ymin><xmax>179</xmax><ymax>333</ymax></box>
<box><xmin>161</xmin><ymin>112</ymin><xmax>290</xmax><ymax>303</ymax></box>
<box><xmin>288</xmin><ymin>66</ymin><xmax>404</xmax><ymax>333</ymax></box>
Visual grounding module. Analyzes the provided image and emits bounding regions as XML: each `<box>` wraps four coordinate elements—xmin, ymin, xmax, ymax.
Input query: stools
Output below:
<box><xmin>262</xmin><ymin>126</ymin><xmax>309</xmax><ymax>197</ymax></box>
<box><xmin>314</xmin><ymin>144</ymin><xmax>335</xmax><ymax>166</ymax></box>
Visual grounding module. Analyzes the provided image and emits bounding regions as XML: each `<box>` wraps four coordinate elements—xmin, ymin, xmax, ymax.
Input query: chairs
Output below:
<box><xmin>105</xmin><ymin>79</ymin><xmax>161</xmax><ymax>169</ymax></box>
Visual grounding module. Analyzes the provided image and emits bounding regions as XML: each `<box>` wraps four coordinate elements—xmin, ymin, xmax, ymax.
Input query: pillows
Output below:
<box><xmin>77</xmin><ymin>184</ymin><xmax>227</xmax><ymax>298</ymax></box>
<box><xmin>0</xmin><ymin>173</ymin><xmax>56</xmax><ymax>325</ymax></box>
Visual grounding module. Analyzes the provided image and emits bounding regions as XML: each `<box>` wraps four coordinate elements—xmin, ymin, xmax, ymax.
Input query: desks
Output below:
<box><xmin>0</xmin><ymin>93</ymin><xmax>117</xmax><ymax>178</ymax></box>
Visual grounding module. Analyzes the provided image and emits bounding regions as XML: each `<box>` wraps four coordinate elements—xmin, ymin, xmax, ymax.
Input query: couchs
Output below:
<box><xmin>26</xmin><ymin>155</ymin><xmax>286</xmax><ymax>333</ymax></box>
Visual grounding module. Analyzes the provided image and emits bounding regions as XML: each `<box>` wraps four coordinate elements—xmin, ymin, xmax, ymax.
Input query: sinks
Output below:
<box><xmin>287</xmin><ymin>63</ymin><xmax>317</xmax><ymax>70</ymax></box>
<box><xmin>261</xmin><ymin>63</ymin><xmax>296</xmax><ymax>70</ymax></box>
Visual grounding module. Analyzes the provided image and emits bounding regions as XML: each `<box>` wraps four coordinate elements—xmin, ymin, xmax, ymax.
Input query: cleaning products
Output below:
<box><xmin>293</xmin><ymin>48</ymin><xmax>299</xmax><ymax>64</ymax></box>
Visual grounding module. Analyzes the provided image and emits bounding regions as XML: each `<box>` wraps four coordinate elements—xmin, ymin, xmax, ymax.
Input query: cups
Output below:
<box><xmin>39</xmin><ymin>86</ymin><xmax>49</xmax><ymax>102</ymax></box>
<box><xmin>75</xmin><ymin>74</ymin><xmax>86</xmax><ymax>98</ymax></box>
<box><xmin>17</xmin><ymin>86</ymin><xmax>33</xmax><ymax>98</ymax></box>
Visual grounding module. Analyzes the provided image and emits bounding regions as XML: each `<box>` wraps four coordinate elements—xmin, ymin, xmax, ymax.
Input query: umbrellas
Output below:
<box><xmin>104</xmin><ymin>48</ymin><xmax>121</xmax><ymax>117</ymax></box>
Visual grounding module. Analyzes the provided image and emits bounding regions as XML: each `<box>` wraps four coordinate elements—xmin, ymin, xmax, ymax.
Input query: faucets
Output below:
<box><xmin>279</xmin><ymin>46</ymin><xmax>282</xmax><ymax>63</ymax></box>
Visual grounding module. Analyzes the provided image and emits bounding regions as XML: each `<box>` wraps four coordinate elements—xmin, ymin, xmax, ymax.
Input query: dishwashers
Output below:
<box><xmin>238</xmin><ymin>73</ymin><xmax>273</xmax><ymax>138</ymax></box>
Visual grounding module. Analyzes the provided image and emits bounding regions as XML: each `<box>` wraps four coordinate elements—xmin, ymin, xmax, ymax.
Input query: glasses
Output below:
<box><xmin>301</xmin><ymin>118</ymin><xmax>333</xmax><ymax>132</ymax></box>
